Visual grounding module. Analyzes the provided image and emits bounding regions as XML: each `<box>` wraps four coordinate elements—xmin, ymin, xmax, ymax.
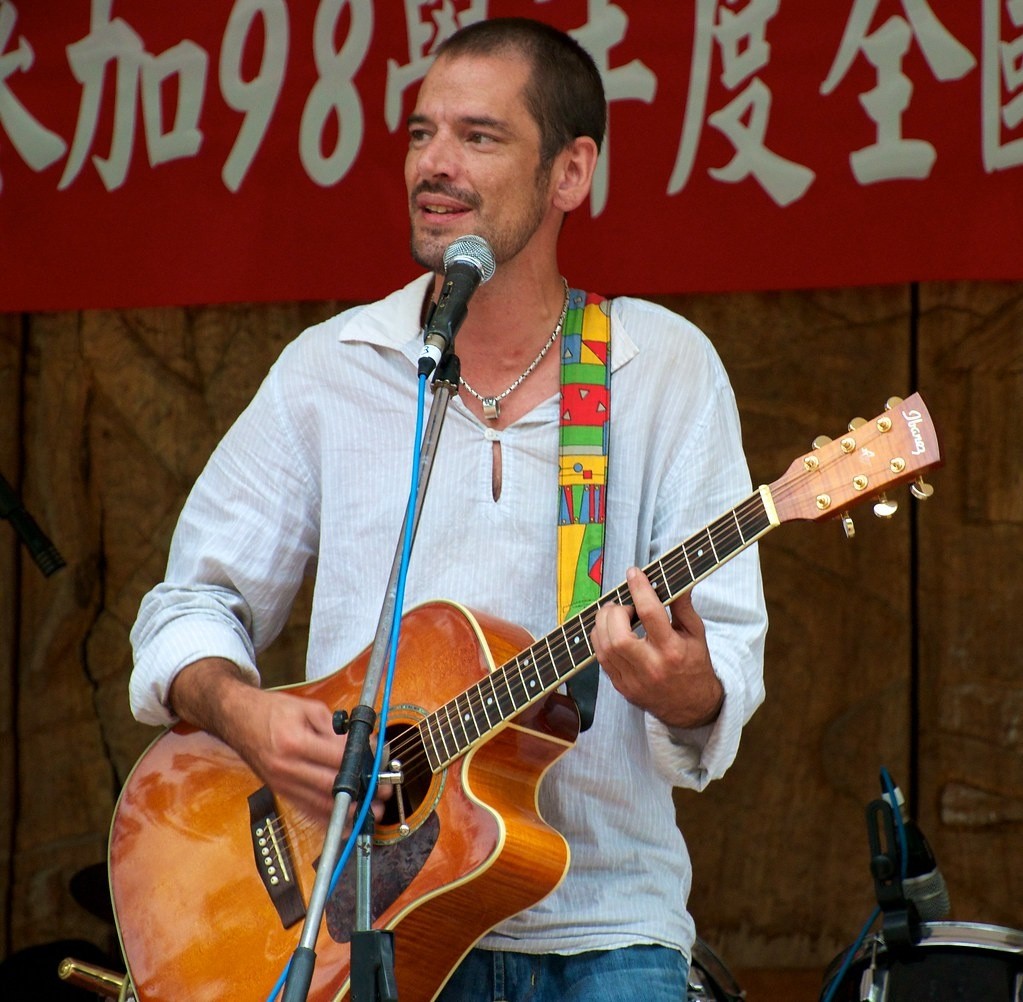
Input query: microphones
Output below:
<box><xmin>418</xmin><ymin>234</ymin><xmax>496</xmax><ymax>379</ymax></box>
<box><xmin>879</xmin><ymin>771</ymin><xmax>950</xmax><ymax>922</ymax></box>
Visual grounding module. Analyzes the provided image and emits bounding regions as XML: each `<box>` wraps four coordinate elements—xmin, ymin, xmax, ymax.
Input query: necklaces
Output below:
<box><xmin>457</xmin><ymin>276</ymin><xmax>571</xmax><ymax>420</ymax></box>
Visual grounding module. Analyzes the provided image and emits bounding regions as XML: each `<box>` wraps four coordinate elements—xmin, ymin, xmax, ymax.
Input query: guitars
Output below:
<box><xmin>104</xmin><ymin>389</ymin><xmax>943</xmax><ymax>1002</ymax></box>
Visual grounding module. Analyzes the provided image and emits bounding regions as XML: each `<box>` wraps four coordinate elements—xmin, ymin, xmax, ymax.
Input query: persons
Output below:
<box><xmin>127</xmin><ymin>17</ymin><xmax>766</xmax><ymax>1002</ymax></box>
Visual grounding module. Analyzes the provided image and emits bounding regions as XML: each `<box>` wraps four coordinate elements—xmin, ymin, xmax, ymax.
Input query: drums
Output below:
<box><xmin>812</xmin><ymin>919</ymin><xmax>1023</xmax><ymax>1002</ymax></box>
